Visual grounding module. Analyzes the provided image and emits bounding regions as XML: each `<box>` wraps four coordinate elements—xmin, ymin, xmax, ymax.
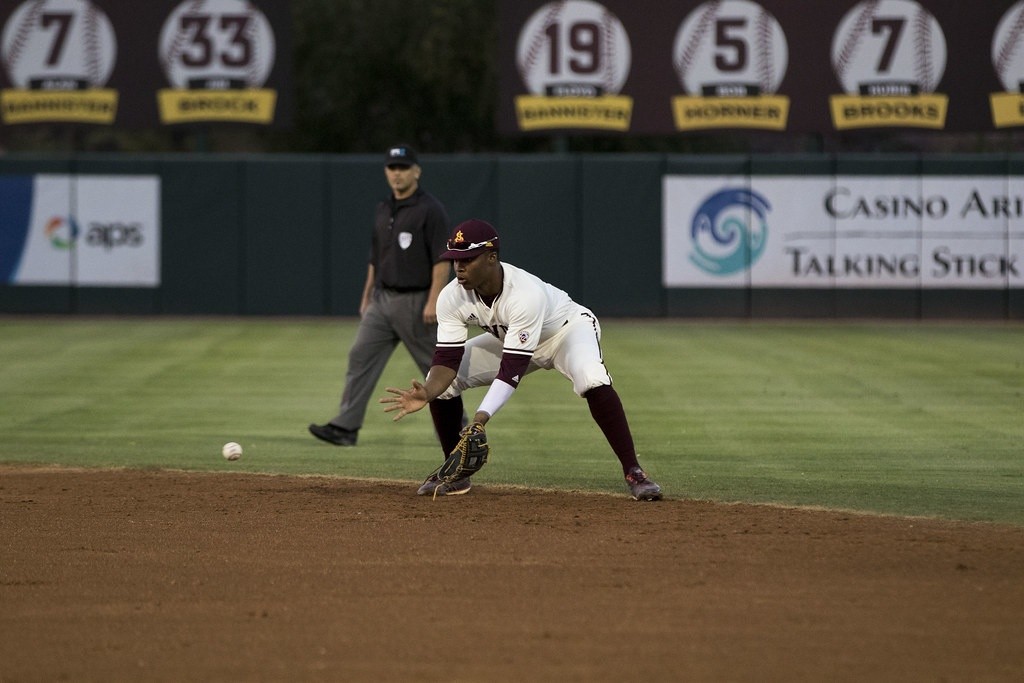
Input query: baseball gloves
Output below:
<box><xmin>437</xmin><ymin>420</ymin><xmax>489</xmax><ymax>484</ymax></box>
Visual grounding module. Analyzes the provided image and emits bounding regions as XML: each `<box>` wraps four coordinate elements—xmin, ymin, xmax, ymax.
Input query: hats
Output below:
<box><xmin>385</xmin><ymin>143</ymin><xmax>418</xmax><ymax>166</ymax></box>
<box><xmin>439</xmin><ymin>218</ymin><xmax>499</xmax><ymax>259</ymax></box>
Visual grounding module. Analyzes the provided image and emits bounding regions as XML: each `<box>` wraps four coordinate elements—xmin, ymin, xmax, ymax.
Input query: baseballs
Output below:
<box><xmin>222</xmin><ymin>441</ymin><xmax>243</xmax><ymax>461</ymax></box>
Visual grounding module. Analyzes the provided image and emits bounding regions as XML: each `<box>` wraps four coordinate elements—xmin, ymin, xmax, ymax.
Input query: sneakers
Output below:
<box><xmin>626</xmin><ymin>466</ymin><xmax>663</xmax><ymax>501</ymax></box>
<box><xmin>309</xmin><ymin>423</ymin><xmax>360</xmax><ymax>446</ymax></box>
<box><xmin>417</xmin><ymin>471</ymin><xmax>472</xmax><ymax>496</ymax></box>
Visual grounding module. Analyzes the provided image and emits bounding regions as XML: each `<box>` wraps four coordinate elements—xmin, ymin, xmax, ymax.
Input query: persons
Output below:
<box><xmin>379</xmin><ymin>216</ymin><xmax>663</xmax><ymax>500</ymax></box>
<box><xmin>309</xmin><ymin>141</ymin><xmax>468</xmax><ymax>463</ymax></box>
